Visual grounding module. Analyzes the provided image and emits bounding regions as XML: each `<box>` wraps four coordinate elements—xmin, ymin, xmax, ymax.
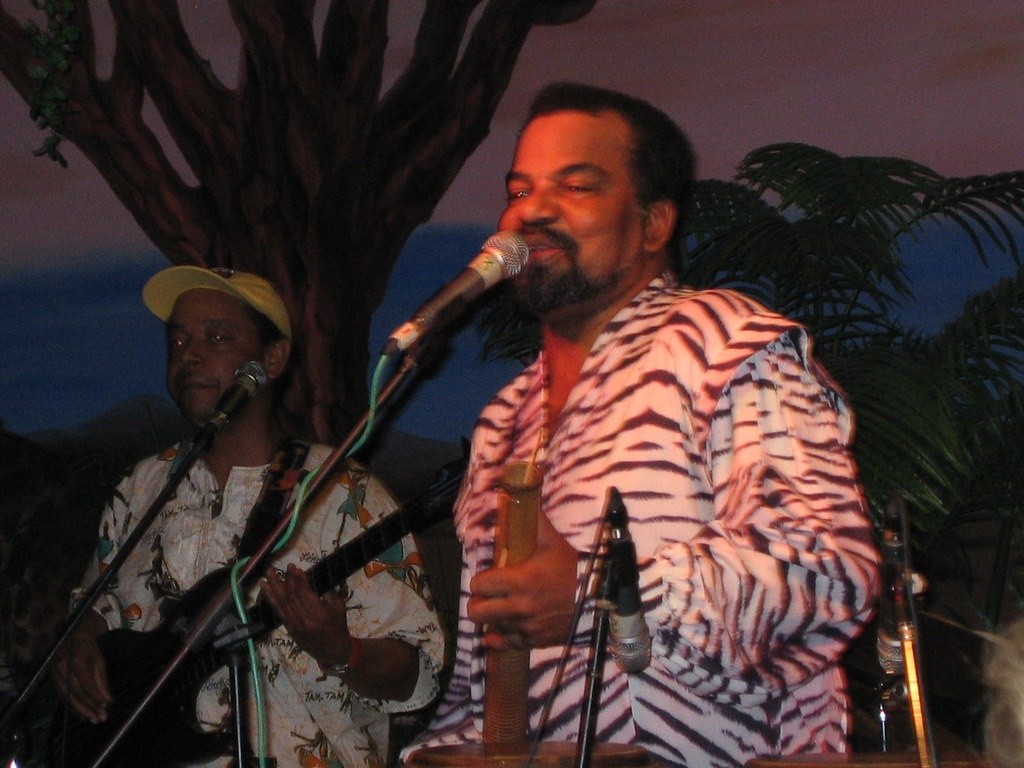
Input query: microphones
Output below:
<box><xmin>196</xmin><ymin>359</ymin><xmax>268</xmax><ymax>444</ymax></box>
<box><xmin>606</xmin><ymin>486</ymin><xmax>652</xmax><ymax>672</ymax></box>
<box><xmin>876</xmin><ymin>496</ymin><xmax>908</xmax><ymax>676</ymax></box>
<box><xmin>379</xmin><ymin>231</ymin><xmax>529</xmax><ymax>358</ymax></box>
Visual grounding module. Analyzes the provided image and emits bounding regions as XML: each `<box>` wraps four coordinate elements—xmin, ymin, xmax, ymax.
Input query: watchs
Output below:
<box><xmin>317</xmin><ymin>639</ymin><xmax>361</xmax><ymax>678</ymax></box>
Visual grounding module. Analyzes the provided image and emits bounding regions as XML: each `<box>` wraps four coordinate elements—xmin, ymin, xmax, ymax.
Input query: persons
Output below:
<box><xmin>54</xmin><ymin>266</ymin><xmax>444</xmax><ymax>768</ymax></box>
<box><xmin>400</xmin><ymin>83</ymin><xmax>881</xmax><ymax>768</ymax></box>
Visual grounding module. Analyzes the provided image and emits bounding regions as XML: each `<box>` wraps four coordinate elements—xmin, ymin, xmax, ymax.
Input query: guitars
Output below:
<box><xmin>13</xmin><ymin>464</ymin><xmax>463</xmax><ymax>768</ymax></box>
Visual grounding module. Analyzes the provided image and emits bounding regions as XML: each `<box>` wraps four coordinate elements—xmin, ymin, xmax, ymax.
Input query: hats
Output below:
<box><xmin>142</xmin><ymin>266</ymin><xmax>292</xmax><ymax>344</ymax></box>
